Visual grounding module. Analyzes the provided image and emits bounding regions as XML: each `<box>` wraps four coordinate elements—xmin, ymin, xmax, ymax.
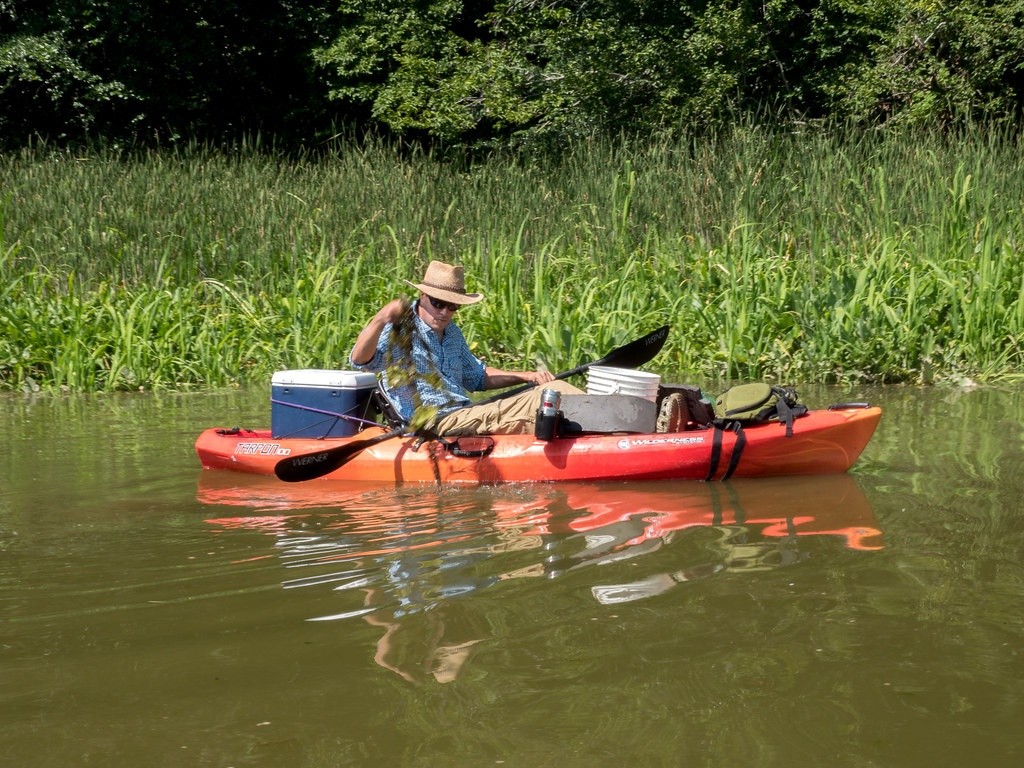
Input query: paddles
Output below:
<box><xmin>273</xmin><ymin>324</ymin><xmax>671</xmax><ymax>484</ymax></box>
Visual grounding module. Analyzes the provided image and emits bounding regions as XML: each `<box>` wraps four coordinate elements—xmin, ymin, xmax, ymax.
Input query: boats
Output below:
<box><xmin>197</xmin><ymin>469</ymin><xmax>886</xmax><ymax>553</ymax></box>
<box><xmin>195</xmin><ymin>403</ymin><xmax>882</xmax><ymax>482</ymax></box>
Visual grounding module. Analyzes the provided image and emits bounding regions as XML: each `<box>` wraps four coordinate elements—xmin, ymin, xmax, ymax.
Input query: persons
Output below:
<box><xmin>348</xmin><ymin>260</ymin><xmax>688</xmax><ymax>437</ymax></box>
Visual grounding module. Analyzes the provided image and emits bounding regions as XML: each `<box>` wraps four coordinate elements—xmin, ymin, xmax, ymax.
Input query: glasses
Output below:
<box><xmin>427</xmin><ymin>294</ymin><xmax>460</xmax><ymax>311</ymax></box>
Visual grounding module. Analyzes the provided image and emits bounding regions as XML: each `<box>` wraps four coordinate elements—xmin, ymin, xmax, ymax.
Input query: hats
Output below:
<box><xmin>404</xmin><ymin>259</ymin><xmax>484</xmax><ymax>305</ymax></box>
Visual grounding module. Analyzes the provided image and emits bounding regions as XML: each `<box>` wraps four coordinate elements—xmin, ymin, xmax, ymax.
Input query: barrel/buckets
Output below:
<box><xmin>587</xmin><ymin>365</ymin><xmax>660</xmax><ymax>404</ymax></box>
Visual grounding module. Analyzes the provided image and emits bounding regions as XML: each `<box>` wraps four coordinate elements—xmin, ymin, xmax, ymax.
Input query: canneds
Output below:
<box><xmin>539</xmin><ymin>388</ymin><xmax>562</xmax><ymax>416</ymax></box>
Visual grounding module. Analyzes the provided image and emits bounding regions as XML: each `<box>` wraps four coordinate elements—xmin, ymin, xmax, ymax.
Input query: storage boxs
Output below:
<box><xmin>271</xmin><ymin>369</ymin><xmax>378</xmax><ymax>439</ymax></box>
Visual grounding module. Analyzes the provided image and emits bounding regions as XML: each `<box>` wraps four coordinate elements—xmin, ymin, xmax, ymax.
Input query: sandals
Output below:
<box><xmin>657</xmin><ymin>393</ymin><xmax>687</xmax><ymax>433</ymax></box>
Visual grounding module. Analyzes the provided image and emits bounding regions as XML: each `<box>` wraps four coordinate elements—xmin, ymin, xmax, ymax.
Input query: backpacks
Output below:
<box><xmin>712</xmin><ymin>382</ymin><xmax>809</xmax><ymax>437</ymax></box>
